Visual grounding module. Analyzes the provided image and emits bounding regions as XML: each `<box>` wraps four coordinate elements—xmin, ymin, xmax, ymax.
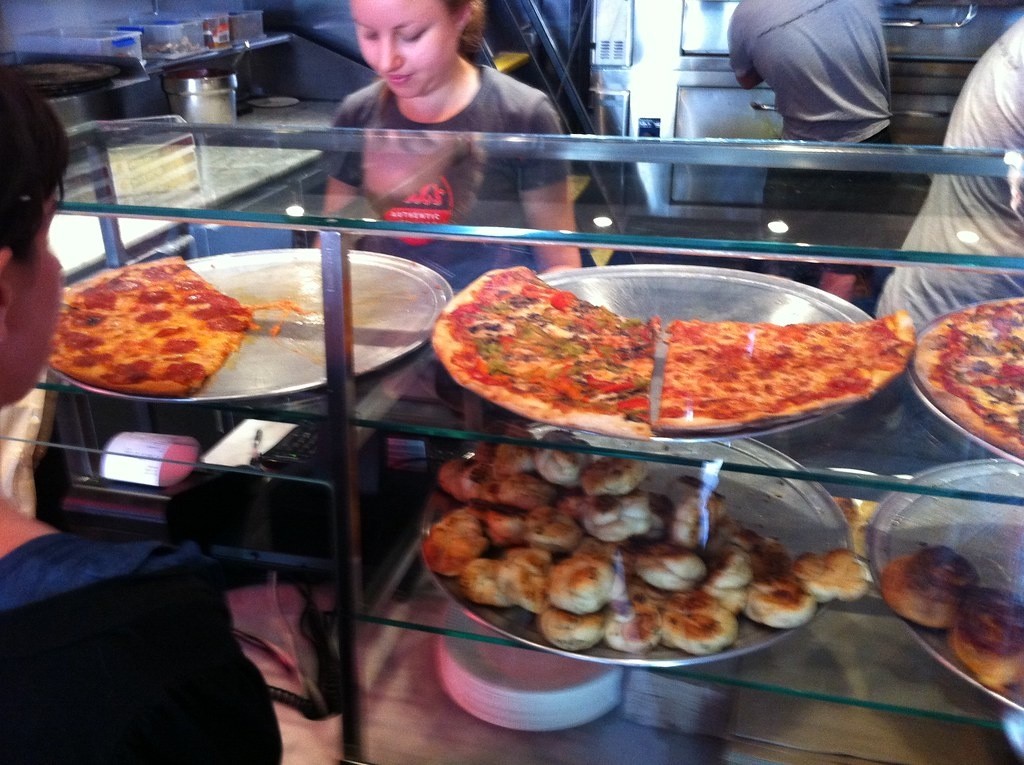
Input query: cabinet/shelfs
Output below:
<box><xmin>1</xmin><ymin>118</ymin><xmax>1023</xmax><ymax>764</ymax></box>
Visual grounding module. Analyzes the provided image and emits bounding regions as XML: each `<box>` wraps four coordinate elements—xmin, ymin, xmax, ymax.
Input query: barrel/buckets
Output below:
<box><xmin>160</xmin><ymin>68</ymin><xmax>238</xmax><ymax>128</ymax></box>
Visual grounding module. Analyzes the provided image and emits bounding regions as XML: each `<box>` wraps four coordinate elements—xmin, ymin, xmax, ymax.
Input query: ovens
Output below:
<box><xmin>673</xmin><ymin>0</ymin><xmax>1024</xmax><ymax>217</ymax></box>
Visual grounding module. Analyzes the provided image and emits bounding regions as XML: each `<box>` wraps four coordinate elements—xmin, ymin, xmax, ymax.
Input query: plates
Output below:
<box><xmin>863</xmin><ymin>459</ymin><xmax>1024</xmax><ymax>711</ymax></box>
<box><xmin>433</xmin><ymin>634</ymin><xmax>622</xmax><ymax>733</ymax></box>
<box><xmin>419</xmin><ymin>437</ymin><xmax>855</xmax><ymax>668</ymax></box>
<box><xmin>247</xmin><ymin>96</ymin><xmax>299</xmax><ymax>107</ymax></box>
<box><xmin>46</xmin><ymin>248</ymin><xmax>455</xmax><ymax>403</ymax></box>
<box><xmin>904</xmin><ymin>299</ymin><xmax>1024</xmax><ymax>468</ymax></box>
<box><xmin>533</xmin><ymin>264</ymin><xmax>876</xmax><ymax>444</ymax></box>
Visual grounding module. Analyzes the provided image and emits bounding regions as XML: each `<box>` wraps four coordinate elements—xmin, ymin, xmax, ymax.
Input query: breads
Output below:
<box><xmin>880</xmin><ymin>544</ymin><xmax>1024</xmax><ymax>689</ymax></box>
<box><xmin>420</xmin><ymin>421</ymin><xmax>871</xmax><ymax>656</ymax></box>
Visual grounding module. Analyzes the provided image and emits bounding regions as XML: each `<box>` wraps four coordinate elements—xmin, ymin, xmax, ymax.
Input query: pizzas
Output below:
<box><xmin>53</xmin><ymin>256</ymin><xmax>254</xmax><ymax>396</ymax></box>
<box><xmin>655</xmin><ymin>309</ymin><xmax>917</xmax><ymax>431</ymax></box>
<box><xmin>432</xmin><ymin>265</ymin><xmax>664</xmax><ymax>438</ymax></box>
<box><xmin>913</xmin><ymin>297</ymin><xmax>1023</xmax><ymax>462</ymax></box>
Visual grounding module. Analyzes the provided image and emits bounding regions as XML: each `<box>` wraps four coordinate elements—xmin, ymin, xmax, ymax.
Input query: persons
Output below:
<box><xmin>877</xmin><ymin>15</ymin><xmax>1024</xmax><ymax>333</ymax></box>
<box><xmin>310</xmin><ymin>0</ymin><xmax>581</xmax><ymax>291</ymax></box>
<box><xmin>726</xmin><ymin>0</ymin><xmax>894</xmax><ymax>299</ymax></box>
<box><xmin>0</xmin><ymin>61</ymin><xmax>286</xmax><ymax>765</ymax></box>
